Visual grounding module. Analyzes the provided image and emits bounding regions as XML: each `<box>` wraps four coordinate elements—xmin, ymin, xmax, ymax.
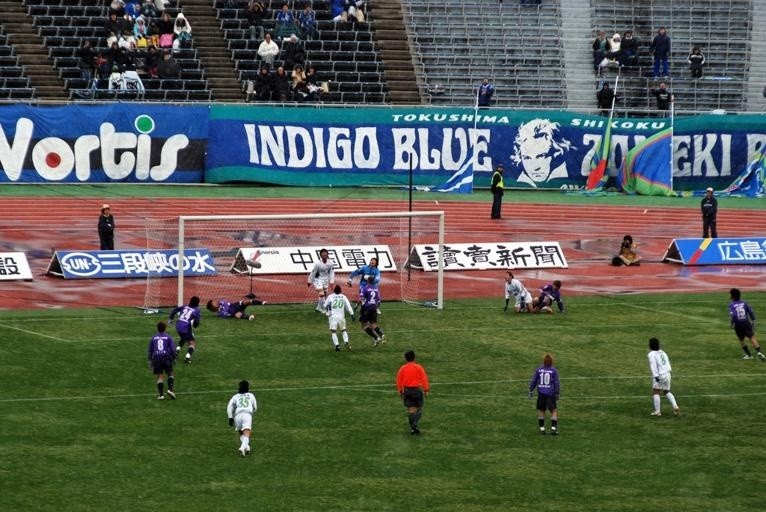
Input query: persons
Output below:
<box><xmin>688</xmin><ymin>47</ymin><xmax>706</xmax><ymax>78</ymax></box>
<box><xmin>396</xmin><ymin>351</ymin><xmax>429</xmax><ymax>435</ymax></box>
<box><xmin>532</xmin><ymin>281</ymin><xmax>565</xmax><ymax>314</ymax></box>
<box><xmin>647</xmin><ymin>337</ymin><xmax>680</xmax><ymax>417</ymax></box>
<box><xmin>619</xmin><ymin>235</ymin><xmax>643</xmax><ymax>268</ymax></box>
<box><xmin>592</xmin><ymin>26</ymin><xmax>671</xmax><ymax>115</ymax></box>
<box><xmin>247</xmin><ymin>0</ymin><xmax>364</xmax><ymax>100</ymax></box>
<box><xmin>148</xmin><ymin>322</ymin><xmax>181</xmax><ymax>402</ymax></box>
<box><xmin>99</xmin><ymin>202</ymin><xmax>116</xmax><ymax>250</ymax></box>
<box><xmin>82</xmin><ymin>0</ymin><xmax>197</xmax><ymax>77</ymax></box>
<box><xmin>480</xmin><ymin>78</ymin><xmax>495</xmax><ymax>109</ymax></box>
<box><xmin>503</xmin><ymin>271</ymin><xmax>536</xmax><ymax>314</ymax></box>
<box><xmin>307</xmin><ymin>248</ymin><xmax>386</xmax><ymax>352</ymax></box>
<box><xmin>728</xmin><ymin>288</ymin><xmax>766</xmax><ymax>361</ymax></box>
<box><xmin>510</xmin><ymin>118</ymin><xmax>578</xmax><ymax>190</ymax></box>
<box><xmin>226</xmin><ymin>380</ymin><xmax>258</xmax><ymax>457</ymax></box>
<box><xmin>528</xmin><ymin>354</ymin><xmax>561</xmax><ymax>435</ymax></box>
<box><xmin>167</xmin><ymin>296</ymin><xmax>202</xmax><ymax>364</ymax></box>
<box><xmin>490</xmin><ymin>163</ymin><xmax>507</xmax><ymax>221</ymax></box>
<box><xmin>207</xmin><ymin>298</ymin><xmax>269</xmax><ymax>321</ymax></box>
<box><xmin>699</xmin><ymin>187</ymin><xmax>718</xmax><ymax>239</ymax></box>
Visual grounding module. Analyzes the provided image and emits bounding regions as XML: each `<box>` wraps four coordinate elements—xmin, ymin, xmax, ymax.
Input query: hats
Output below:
<box><xmin>706</xmin><ymin>187</ymin><xmax>715</xmax><ymax>193</ymax></box>
<box><xmin>100</xmin><ymin>204</ymin><xmax>110</xmax><ymax>210</ymax></box>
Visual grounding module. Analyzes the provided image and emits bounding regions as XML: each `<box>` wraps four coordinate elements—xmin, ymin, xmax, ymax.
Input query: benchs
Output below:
<box><xmin>0</xmin><ymin>1</ymin><xmax>766</xmax><ymax>121</ymax></box>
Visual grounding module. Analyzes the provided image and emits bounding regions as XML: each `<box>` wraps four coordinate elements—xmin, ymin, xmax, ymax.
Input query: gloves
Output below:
<box><xmin>554</xmin><ymin>395</ymin><xmax>560</xmax><ymax>401</ymax></box>
<box><xmin>528</xmin><ymin>392</ymin><xmax>533</xmax><ymax>400</ymax></box>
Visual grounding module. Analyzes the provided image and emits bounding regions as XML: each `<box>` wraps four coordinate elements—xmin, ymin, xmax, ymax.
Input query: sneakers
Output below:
<box><xmin>540</xmin><ymin>429</ymin><xmax>559</xmax><ymax>435</ymax></box>
<box><xmin>371</xmin><ymin>334</ymin><xmax>387</xmax><ymax>347</ymax></box>
<box><xmin>335</xmin><ymin>342</ymin><xmax>352</xmax><ymax>352</ymax></box>
<box><xmin>246</xmin><ymin>448</ymin><xmax>250</xmax><ymax>454</ymax></box>
<box><xmin>185</xmin><ymin>358</ymin><xmax>191</xmax><ymax>365</ymax></box>
<box><xmin>167</xmin><ymin>390</ymin><xmax>176</xmax><ymax>399</ymax></box>
<box><xmin>249</xmin><ymin>315</ymin><xmax>255</xmax><ymax>321</ymax></box>
<box><xmin>757</xmin><ymin>352</ymin><xmax>766</xmax><ymax>362</ymax></box>
<box><xmin>742</xmin><ymin>355</ymin><xmax>754</xmax><ymax>360</ymax></box>
<box><xmin>411</xmin><ymin>425</ymin><xmax>420</xmax><ymax>435</ymax></box>
<box><xmin>377</xmin><ymin>309</ymin><xmax>382</xmax><ymax>315</ymax></box>
<box><xmin>239</xmin><ymin>448</ymin><xmax>245</xmax><ymax>456</ymax></box>
<box><xmin>157</xmin><ymin>395</ymin><xmax>165</xmax><ymax>400</ymax></box>
<box><xmin>674</xmin><ymin>407</ymin><xmax>680</xmax><ymax>417</ymax></box>
<box><xmin>651</xmin><ymin>411</ymin><xmax>662</xmax><ymax>416</ymax></box>
<box><xmin>262</xmin><ymin>300</ymin><xmax>268</xmax><ymax>306</ymax></box>
<box><xmin>315</xmin><ymin>306</ymin><xmax>326</xmax><ymax>313</ymax></box>
